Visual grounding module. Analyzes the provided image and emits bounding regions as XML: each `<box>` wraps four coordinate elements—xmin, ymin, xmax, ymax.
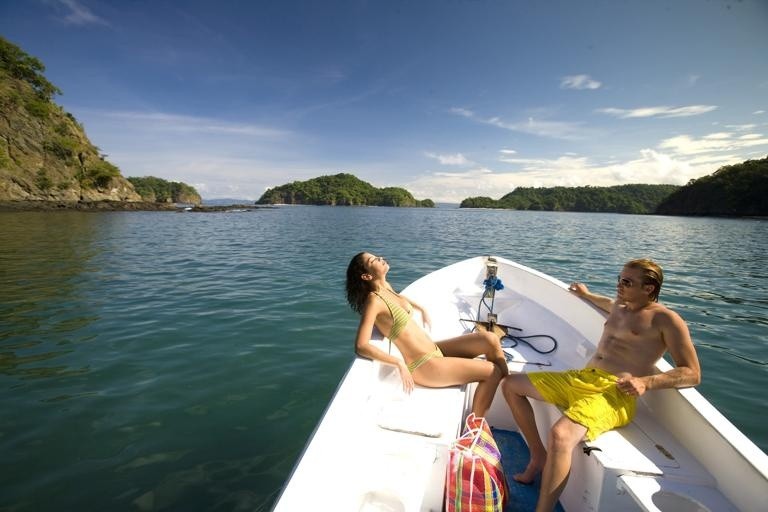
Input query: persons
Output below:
<box><xmin>499</xmin><ymin>259</ymin><xmax>701</xmax><ymax>512</ymax></box>
<box><xmin>345</xmin><ymin>250</ymin><xmax>512</xmax><ymax>417</ymax></box>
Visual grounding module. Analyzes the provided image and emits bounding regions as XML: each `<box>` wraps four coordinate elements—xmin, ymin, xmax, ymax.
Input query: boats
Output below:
<box><xmin>271</xmin><ymin>255</ymin><xmax>768</xmax><ymax>511</ymax></box>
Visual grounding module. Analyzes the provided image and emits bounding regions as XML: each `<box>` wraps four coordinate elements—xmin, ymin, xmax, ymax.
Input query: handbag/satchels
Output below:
<box><xmin>444</xmin><ymin>412</ymin><xmax>512</xmax><ymax>512</ymax></box>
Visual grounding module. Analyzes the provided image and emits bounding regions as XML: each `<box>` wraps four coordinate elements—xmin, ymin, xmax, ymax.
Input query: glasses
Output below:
<box><xmin>617</xmin><ymin>275</ymin><xmax>633</xmax><ymax>288</ymax></box>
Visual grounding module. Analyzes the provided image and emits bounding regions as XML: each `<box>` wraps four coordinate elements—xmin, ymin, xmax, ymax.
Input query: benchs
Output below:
<box><xmin>521</xmin><ymin>342</ymin><xmax>734</xmax><ymax>512</ymax></box>
<box><xmin>319</xmin><ymin>340</ymin><xmax>470</xmax><ymax>512</ymax></box>
